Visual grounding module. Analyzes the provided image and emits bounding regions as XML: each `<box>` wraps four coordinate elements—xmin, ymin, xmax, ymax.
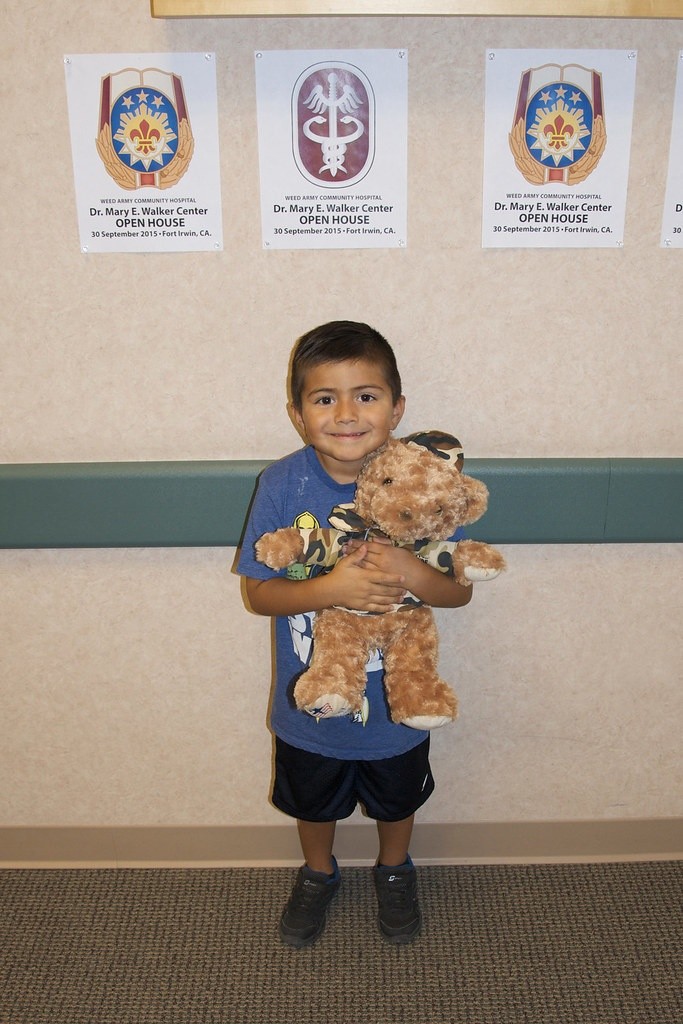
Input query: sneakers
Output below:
<box><xmin>280</xmin><ymin>855</ymin><xmax>341</xmax><ymax>946</ymax></box>
<box><xmin>373</xmin><ymin>854</ymin><xmax>422</xmax><ymax>945</ymax></box>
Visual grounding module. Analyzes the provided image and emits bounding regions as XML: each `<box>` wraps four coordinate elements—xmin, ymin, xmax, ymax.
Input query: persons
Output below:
<box><xmin>232</xmin><ymin>321</ymin><xmax>473</xmax><ymax>946</ymax></box>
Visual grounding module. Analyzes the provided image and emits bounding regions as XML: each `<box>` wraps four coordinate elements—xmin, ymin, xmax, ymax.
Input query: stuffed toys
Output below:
<box><xmin>254</xmin><ymin>430</ymin><xmax>504</xmax><ymax>730</ymax></box>
